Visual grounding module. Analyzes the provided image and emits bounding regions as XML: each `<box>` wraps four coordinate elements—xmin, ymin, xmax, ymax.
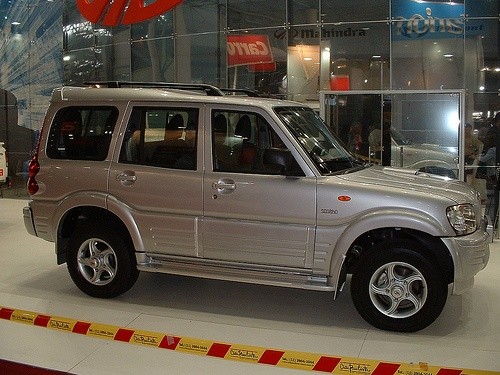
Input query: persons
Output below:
<box><xmin>464</xmin><ymin>109</ymin><xmax>500</xmax><ymax>228</ymax></box>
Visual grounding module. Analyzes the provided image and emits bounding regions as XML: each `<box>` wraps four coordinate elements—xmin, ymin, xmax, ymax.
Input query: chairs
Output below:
<box><xmin>233</xmin><ymin>115</ymin><xmax>260</xmax><ymax>173</ymax></box>
<box><xmin>157</xmin><ymin>114</ymin><xmax>191</xmax><ymax>169</ymax></box>
<box><xmin>214</xmin><ymin>114</ymin><xmax>235</xmax><ymax>173</ymax></box>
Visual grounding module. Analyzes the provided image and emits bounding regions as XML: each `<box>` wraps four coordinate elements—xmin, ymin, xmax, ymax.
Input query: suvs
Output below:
<box><xmin>385</xmin><ymin>122</ymin><xmax>455</xmax><ymax>179</ymax></box>
<box><xmin>23</xmin><ymin>78</ymin><xmax>494</xmax><ymax>333</ymax></box>
